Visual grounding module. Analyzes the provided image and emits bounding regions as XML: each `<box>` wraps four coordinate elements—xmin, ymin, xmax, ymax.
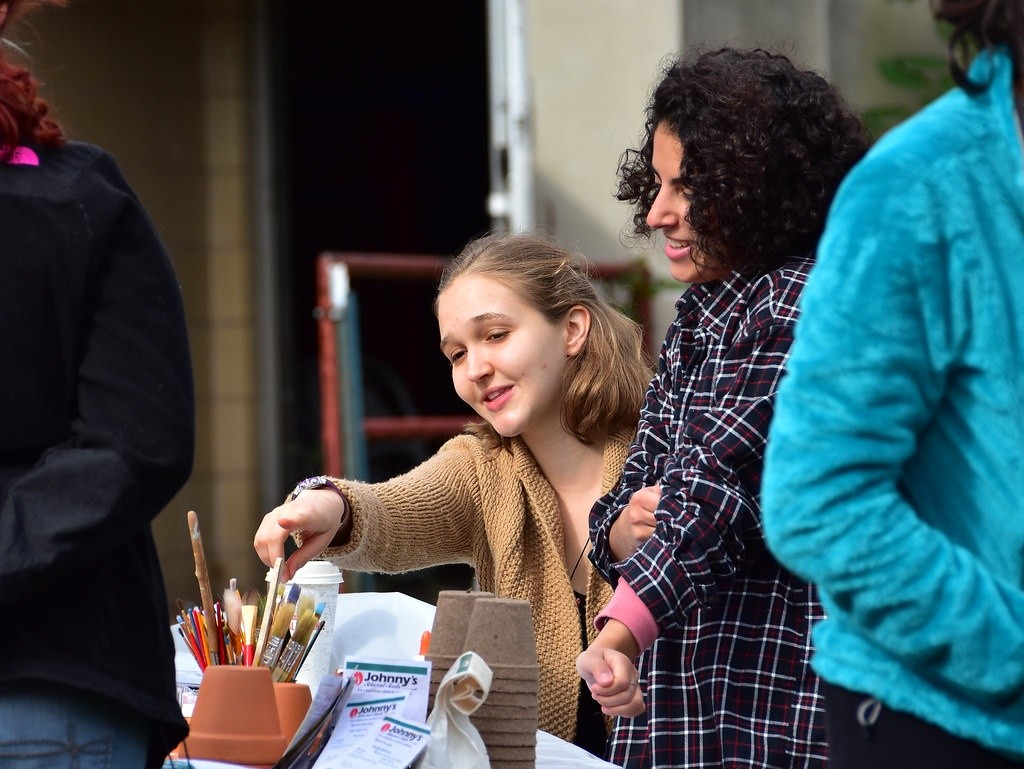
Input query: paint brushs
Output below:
<box><xmin>175</xmin><ymin>510</ymin><xmax>327</xmax><ymax>684</ymax></box>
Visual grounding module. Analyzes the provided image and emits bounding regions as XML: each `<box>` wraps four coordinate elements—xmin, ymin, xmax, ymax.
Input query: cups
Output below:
<box><xmin>181</xmin><ymin>664</ymin><xmax>287</xmax><ymax>763</ymax></box>
<box><xmin>427</xmin><ymin>590</ymin><xmax>539</xmax><ymax>769</ymax></box>
<box><xmin>272</xmin><ymin>681</ymin><xmax>312</xmax><ymax>746</ymax></box>
<box><xmin>282</xmin><ymin>559</ymin><xmax>343</xmax><ymax>701</ymax></box>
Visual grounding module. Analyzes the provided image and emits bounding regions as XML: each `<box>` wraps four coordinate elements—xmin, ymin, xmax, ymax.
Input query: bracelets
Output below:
<box><xmin>291</xmin><ymin>478</ymin><xmax>347</xmax><ymax>528</ymax></box>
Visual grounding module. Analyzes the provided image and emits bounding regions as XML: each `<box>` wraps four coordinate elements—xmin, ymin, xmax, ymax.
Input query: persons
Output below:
<box><xmin>761</xmin><ymin>0</ymin><xmax>1023</xmax><ymax>769</ymax></box>
<box><xmin>575</xmin><ymin>48</ymin><xmax>872</xmax><ymax>768</ymax></box>
<box><xmin>254</xmin><ymin>233</ymin><xmax>659</xmax><ymax>757</ymax></box>
<box><xmin>2</xmin><ymin>2</ymin><xmax>193</xmax><ymax>769</ymax></box>
